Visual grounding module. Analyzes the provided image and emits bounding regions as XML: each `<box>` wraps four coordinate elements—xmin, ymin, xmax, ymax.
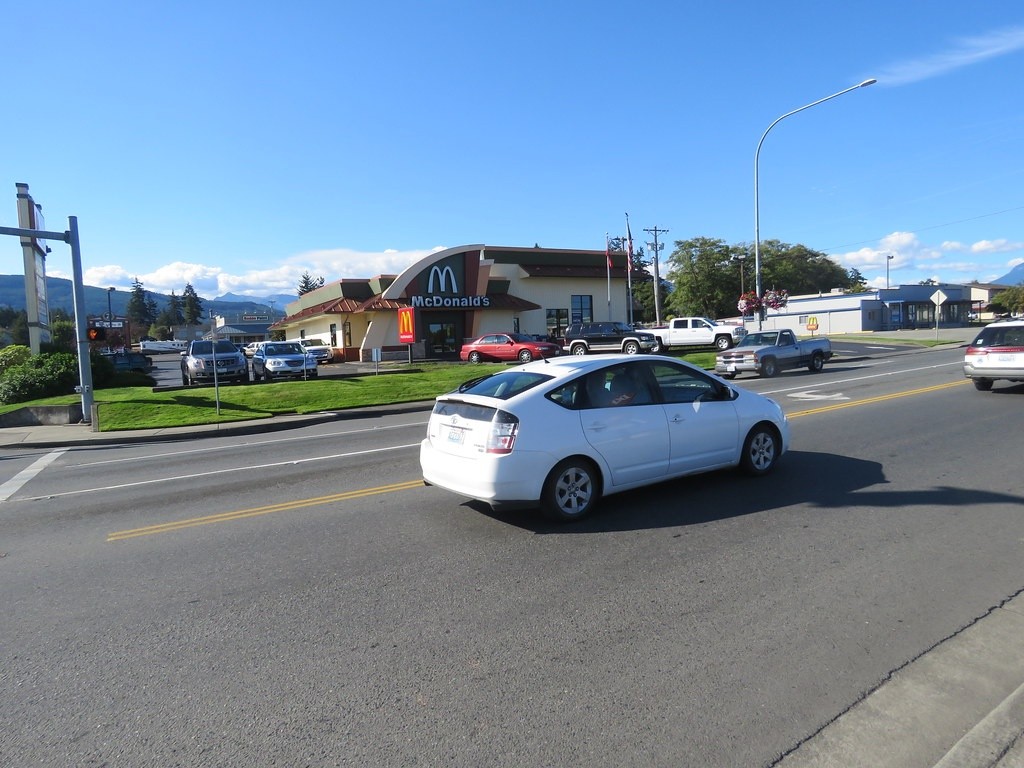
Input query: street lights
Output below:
<box><xmin>887</xmin><ymin>254</ymin><xmax>893</xmax><ymax>289</ymax></box>
<box><xmin>108</xmin><ymin>287</ymin><xmax>115</xmax><ymax>328</ymax></box>
<box><xmin>756</xmin><ymin>78</ymin><xmax>877</xmax><ymax>323</ymax></box>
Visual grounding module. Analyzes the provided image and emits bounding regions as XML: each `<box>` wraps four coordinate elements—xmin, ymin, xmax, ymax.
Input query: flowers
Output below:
<box><xmin>736</xmin><ymin>287</ymin><xmax>788</xmax><ymax>314</ymax></box>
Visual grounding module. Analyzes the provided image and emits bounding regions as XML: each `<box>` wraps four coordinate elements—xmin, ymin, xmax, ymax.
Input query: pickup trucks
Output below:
<box><xmin>714</xmin><ymin>329</ymin><xmax>838</xmax><ymax>380</ymax></box>
<box><xmin>634</xmin><ymin>317</ymin><xmax>748</xmax><ymax>353</ymax></box>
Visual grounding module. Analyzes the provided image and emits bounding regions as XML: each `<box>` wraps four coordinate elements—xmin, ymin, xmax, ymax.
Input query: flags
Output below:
<box><xmin>628</xmin><ymin>226</ymin><xmax>635</xmax><ymax>271</ymax></box>
<box><xmin>606</xmin><ymin>247</ymin><xmax>613</xmax><ymax>269</ymax></box>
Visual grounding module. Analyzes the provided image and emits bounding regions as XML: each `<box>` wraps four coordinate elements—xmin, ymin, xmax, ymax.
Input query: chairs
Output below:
<box><xmin>753</xmin><ymin>335</ymin><xmax>764</xmax><ymax>345</ymax></box>
<box><xmin>571</xmin><ymin>390</ymin><xmax>591</xmax><ymax>409</ymax></box>
<box><xmin>642</xmin><ymin>366</ymin><xmax>666</xmax><ymax>404</ymax></box>
<box><xmin>610</xmin><ymin>375</ymin><xmax>633</xmax><ymax>394</ymax></box>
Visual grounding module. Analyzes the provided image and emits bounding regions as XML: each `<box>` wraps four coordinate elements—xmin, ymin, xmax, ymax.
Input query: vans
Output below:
<box><xmin>242</xmin><ymin>341</ymin><xmax>271</xmax><ymax>356</ymax></box>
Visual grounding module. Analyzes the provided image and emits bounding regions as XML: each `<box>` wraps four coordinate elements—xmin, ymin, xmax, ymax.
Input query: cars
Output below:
<box><xmin>963</xmin><ymin>316</ymin><xmax>1024</xmax><ymax>391</ymax></box>
<box><xmin>433</xmin><ymin>345</ymin><xmax>455</xmax><ymax>353</ymax></box>
<box><xmin>460</xmin><ymin>332</ymin><xmax>561</xmax><ymax>364</ymax></box>
<box><xmin>419</xmin><ymin>353</ymin><xmax>792</xmax><ymax>519</ymax></box>
<box><xmin>968</xmin><ymin>311</ymin><xmax>1010</xmax><ymax>319</ymax></box>
<box><xmin>251</xmin><ymin>343</ymin><xmax>318</xmax><ymax>381</ymax></box>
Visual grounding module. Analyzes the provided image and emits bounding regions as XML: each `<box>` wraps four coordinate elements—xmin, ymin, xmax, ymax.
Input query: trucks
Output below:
<box><xmin>140</xmin><ymin>340</ymin><xmax>188</xmax><ymax>355</ymax></box>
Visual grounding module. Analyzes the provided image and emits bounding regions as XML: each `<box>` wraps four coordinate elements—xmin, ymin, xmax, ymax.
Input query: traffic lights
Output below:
<box><xmin>88</xmin><ymin>327</ymin><xmax>106</xmax><ymax>341</ymax></box>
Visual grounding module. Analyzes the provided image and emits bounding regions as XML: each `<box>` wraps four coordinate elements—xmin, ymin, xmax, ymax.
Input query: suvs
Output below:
<box><xmin>180</xmin><ymin>339</ymin><xmax>249</xmax><ymax>387</ymax></box>
<box><xmin>294</xmin><ymin>340</ymin><xmax>334</xmax><ymax>365</ymax></box>
<box><xmin>563</xmin><ymin>321</ymin><xmax>658</xmax><ymax>356</ymax></box>
